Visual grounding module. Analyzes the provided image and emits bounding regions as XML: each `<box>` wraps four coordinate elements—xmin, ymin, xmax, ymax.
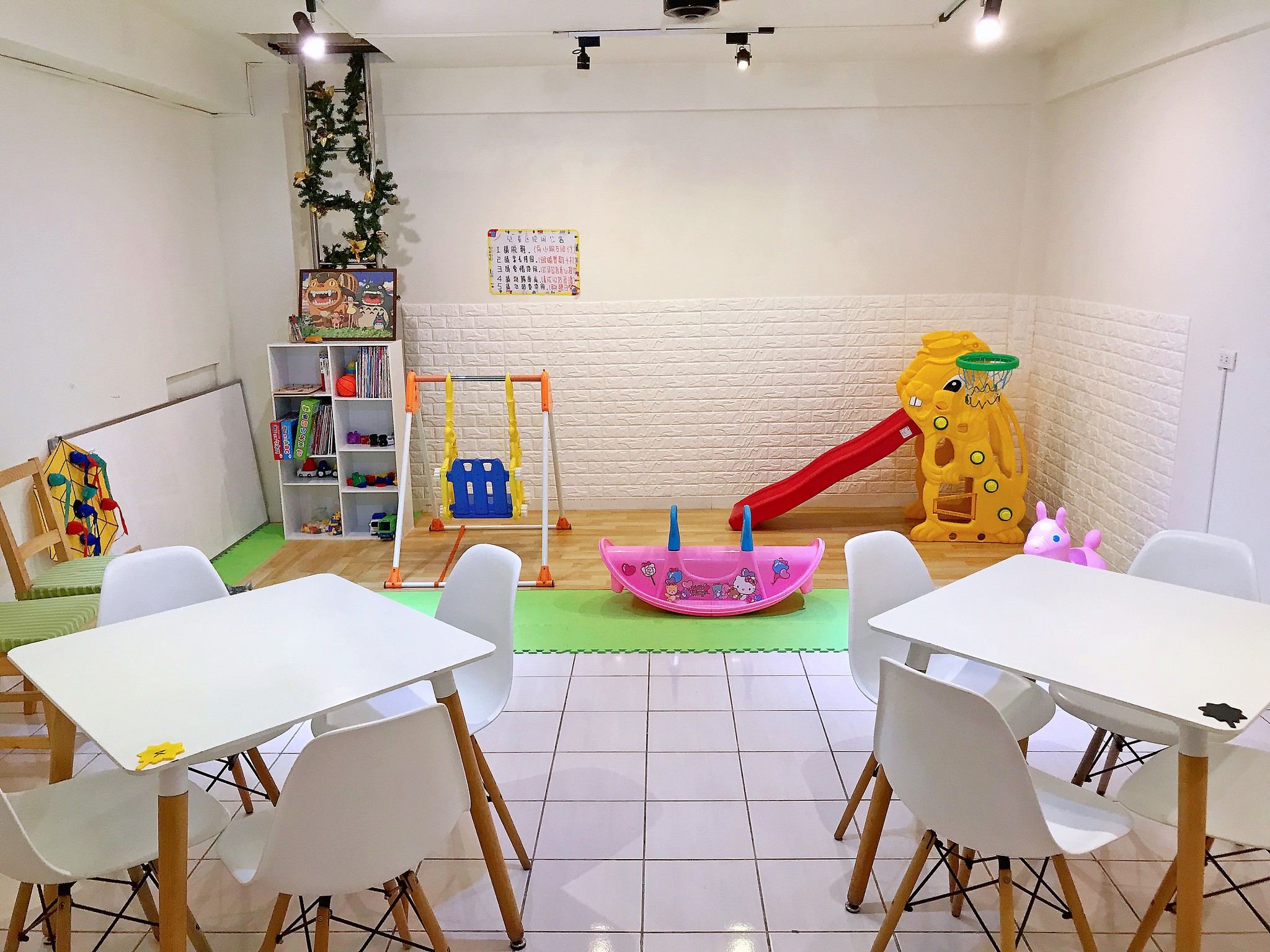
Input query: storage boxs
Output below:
<box><xmin>292</xmin><ymin>398</ymin><xmax>321</xmax><ymax>462</ymax></box>
<box><xmin>270</xmin><ymin>416</ymin><xmax>291</xmax><ymax>461</ymax></box>
<box><xmin>280</xmin><ymin>415</ymin><xmax>299</xmax><ymax>461</ymax></box>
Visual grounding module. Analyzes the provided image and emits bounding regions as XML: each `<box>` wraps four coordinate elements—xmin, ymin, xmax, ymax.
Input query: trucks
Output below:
<box><xmin>378</xmin><ymin>515</ymin><xmax>397</xmax><ymax>540</ymax></box>
<box><xmin>369</xmin><ymin>512</ymin><xmax>387</xmax><ymax>536</ymax></box>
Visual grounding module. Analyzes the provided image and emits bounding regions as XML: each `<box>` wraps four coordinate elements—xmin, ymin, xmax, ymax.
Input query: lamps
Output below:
<box><xmin>725</xmin><ymin>25</ymin><xmax>777</xmax><ymax>70</ymax></box>
<box><xmin>291</xmin><ymin>0</ymin><xmax>329</xmax><ymax>61</ymax></box>
<box><xmin>937</xmin><ymin>0</ymin><xmax>1004</xmax><ymax>45</ymax></box>
<box><xmin>571</xmin><ymin>34</ymin><xmax>602</xmax><ymax>73</ymax></box>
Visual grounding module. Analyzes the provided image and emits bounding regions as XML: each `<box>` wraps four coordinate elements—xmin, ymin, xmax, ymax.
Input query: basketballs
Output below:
<box><xmin>337</xmin><ymin>376</ymin><xmax>356</xmax><ymax>396</ymax></box>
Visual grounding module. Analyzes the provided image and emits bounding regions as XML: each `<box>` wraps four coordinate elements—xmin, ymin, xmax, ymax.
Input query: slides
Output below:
<box><xmin>728</xmin><ymin>407</ymin><xmax>922</xmax><ymax>531</ymax></box>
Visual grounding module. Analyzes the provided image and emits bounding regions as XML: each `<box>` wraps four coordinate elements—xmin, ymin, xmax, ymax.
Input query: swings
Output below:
<box><xmin>440</xmin><ymin>371</ymin><xmax>528</xmax><ymax>519</ymax></box>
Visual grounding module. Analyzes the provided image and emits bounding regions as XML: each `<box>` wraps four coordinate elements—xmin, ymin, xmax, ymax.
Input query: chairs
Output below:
<box><xmin>95</xmin><ymin>542</ymin><xmax>283</xmax><ymax>814</ymax></box>
<box><xmin>1116</xmin><ymin>742</ymin><xmax>1270</xmax><ymax>952</ymax></box>
<box><xmin>829</xmin><ymin>528</ymin><xmax>1057</xmax><ymax>915</ymax></box>
<box><xmin>870</xmin><ymin>652</ymin><xmax>1137</xmax><ymax>952</ymax></box>
<box><xmin>1070</xmin><ymin>528</ymin><xmax>1264</xmax><ymax>798</ymax></box>
<box><xmin>0</xmin><ymin>592</ymin><xmax>101</xmax><ymax>787</ymax></box>
<box><xmin>0</xmin><ymin>457</ymin><xmax>133</xmax><ymax>718</ymax></box>
<box><xmin>0</xmin><ymin>767</ymin><xmax>234</xmax><ymax>952</ymax></box>
<box><xmin>216</xmin><ymin>699</ymin><xmax>473</xmax><ymax>952</ymax></box>
<box><xmin>308</xmin><ymin>541</ymin><xmax>534</xmax><ymax>875</ymax></box>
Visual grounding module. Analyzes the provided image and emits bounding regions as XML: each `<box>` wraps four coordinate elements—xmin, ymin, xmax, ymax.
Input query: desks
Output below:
<box><xmin>5</xmin><ymin>570</ymin><xmax>530</xmax><ymax>952</ymax></box>
<box><xmin>864</xmin><ymin>551</ymin><xmax>1270</xmax><ymax>952</ymax></box>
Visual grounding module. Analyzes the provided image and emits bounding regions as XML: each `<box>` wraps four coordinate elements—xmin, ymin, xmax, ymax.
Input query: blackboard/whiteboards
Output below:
<box><xmin>48</xmin><ymin>380</ymin><xmax>270</xmax><ymax>582</ymax></box>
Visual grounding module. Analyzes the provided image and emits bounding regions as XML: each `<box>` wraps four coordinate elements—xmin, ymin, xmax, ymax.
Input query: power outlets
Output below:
<box><xmin>1216</xmin><ymin>349</ymin><xmax>1238</xmax><ymax>371</ymax></box>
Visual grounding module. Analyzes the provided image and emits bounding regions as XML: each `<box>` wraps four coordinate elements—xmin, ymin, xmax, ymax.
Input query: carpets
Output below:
<box><xmin>211</xmin><ymin>517</ymin><xmax>849</xmax><ymax>659</ymax></box>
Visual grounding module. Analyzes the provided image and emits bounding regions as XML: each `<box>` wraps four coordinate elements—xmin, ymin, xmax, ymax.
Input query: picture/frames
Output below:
<box><xmin>295</xmin><ymin>266</ymin><xmax>398</xmax><ymax>343</ymax></box>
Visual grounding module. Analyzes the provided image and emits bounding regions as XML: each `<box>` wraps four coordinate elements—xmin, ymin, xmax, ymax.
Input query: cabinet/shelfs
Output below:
<box><xmin>264</xmin><ymin>338</ymin><xmax>415</xmax><ymax>541</ymax></box>
